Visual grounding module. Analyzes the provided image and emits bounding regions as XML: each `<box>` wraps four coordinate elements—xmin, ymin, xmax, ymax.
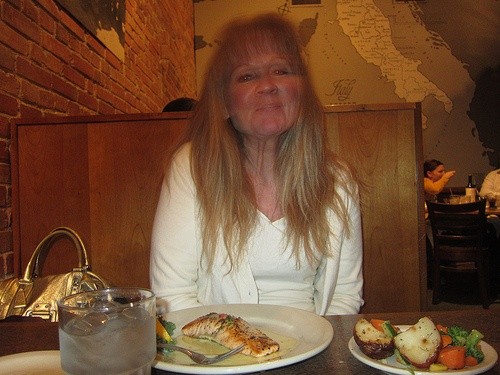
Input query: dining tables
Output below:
<box><xmin>0</xmin><ymin>308</ymin><xmax>500</xmax><ymax>375</ymax></box>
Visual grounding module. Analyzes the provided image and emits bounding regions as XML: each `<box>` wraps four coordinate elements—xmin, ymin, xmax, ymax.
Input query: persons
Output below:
<box><xmin>160</xmin><ymin>97</ymin><xmax>198</xmax><ymax>111</ymax></box>
<box><xmin>480</xmin><ymin>168</ymin><xmax>500</xmax><ymax>208</ymax></box>
<box><xmin>423</xmin><ymin>160</ymin><xmax>456</xmax><ymax>195</ymax></box>
<box><xmin>148</xmin><ymin>11</ymin><xmax>365</xmax><ymax>317</ymax></box>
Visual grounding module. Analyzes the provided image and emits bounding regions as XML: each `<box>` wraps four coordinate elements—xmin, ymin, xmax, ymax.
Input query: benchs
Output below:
<box><xmin>11</xmin><ymin>99</ymin><xmax>432</xmax><ymax>312</ymax></box>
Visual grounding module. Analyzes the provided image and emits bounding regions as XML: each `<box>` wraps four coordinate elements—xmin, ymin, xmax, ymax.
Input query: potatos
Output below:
<box><xmin>393</xmin><ymin>317</ymin><xmax>442</xmax><ymax>368</ymax></box>
<box><xmin>353</xmin><ymin>318</ymin><xmax>395</xmax><ymax>359</ymax></box>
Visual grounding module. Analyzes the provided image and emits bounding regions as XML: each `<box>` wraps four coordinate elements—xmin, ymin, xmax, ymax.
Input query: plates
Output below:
<box><xmin>0</xmin><ymin>350</ymin><xmax>66</xmax><ymax>375</ymax></box>
<box><xmin>156</xmin><ymin>303</ymin><xmax>335</xmax><ymax>375</ymax></box>
<box><xmin>348</xmin><ymin>324</ymin><xmax>498</xmax><ymax>375</ymax></box>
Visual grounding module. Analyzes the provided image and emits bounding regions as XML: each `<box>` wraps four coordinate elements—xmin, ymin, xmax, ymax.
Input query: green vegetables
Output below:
<box><xmin>156</xmin><ymin>316</ymin><xmax>177</xmax><ymax>353</ymax></box>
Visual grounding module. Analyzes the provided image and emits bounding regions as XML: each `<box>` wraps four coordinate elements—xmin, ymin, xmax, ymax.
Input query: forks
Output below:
<box><xmin>157</xmin><ymin>341</ymin><xmax>246</xmax><ymax>366</ymax></box>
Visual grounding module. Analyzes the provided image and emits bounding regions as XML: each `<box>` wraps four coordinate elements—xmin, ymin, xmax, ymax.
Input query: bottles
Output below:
<box><xmin>466</xmin><ymin>175</ymin><xmax>474</xmax><ymax>188</ymax></box>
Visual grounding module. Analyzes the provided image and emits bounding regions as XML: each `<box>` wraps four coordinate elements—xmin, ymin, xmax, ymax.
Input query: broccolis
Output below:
<box><xmin>439</xmin><ymin>325</ymin><xmax>485</xmax><ymax>360</ymax></box>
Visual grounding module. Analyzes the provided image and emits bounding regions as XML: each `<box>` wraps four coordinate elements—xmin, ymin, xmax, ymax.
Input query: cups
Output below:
<box><xmin>465</xmin><ymin>187</ymin><xmax>477</xmax><ymax>203</ymax></box>
<box><xmin>450</xmin><ymin>195</ymin><xmax>460</xmax><ymax>204</ymax></box>
<box><xmin>483</xmin><ymin>192</ymin><xmax>500</xmax><ymax>208</ymax></box>
<box><xmin>57</xmin><ymin>286</ymin><xmax>157</xmax><ymax>375</ymax></box>
<box><xmin>460</xmin><ymin>195</ymin><xmax>471</xmax><ymax>204</ymax></box>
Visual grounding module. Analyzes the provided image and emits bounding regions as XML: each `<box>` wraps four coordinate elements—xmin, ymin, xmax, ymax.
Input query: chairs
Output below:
<box><xmin>424</xmin><ymin>199</ymin><xmax>494</xmax><ymax>314</ymax></box>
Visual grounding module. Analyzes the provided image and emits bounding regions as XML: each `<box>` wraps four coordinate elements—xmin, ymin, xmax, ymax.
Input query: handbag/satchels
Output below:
<box><xmin>0</xmin><ymin>226</ymin><xmax>115</xmax><ymax>324</ymax></box>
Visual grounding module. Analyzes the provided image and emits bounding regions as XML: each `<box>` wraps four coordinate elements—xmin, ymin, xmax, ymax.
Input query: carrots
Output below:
<box><xmin>370</xmin><ymin>319</ymin><xmax>399</xmax><ymax>334</ymax></box>
<box><xmin>435</xmin><ymin>324</ymin><xmax>478</xmax><ymax>371</ymax></box>
